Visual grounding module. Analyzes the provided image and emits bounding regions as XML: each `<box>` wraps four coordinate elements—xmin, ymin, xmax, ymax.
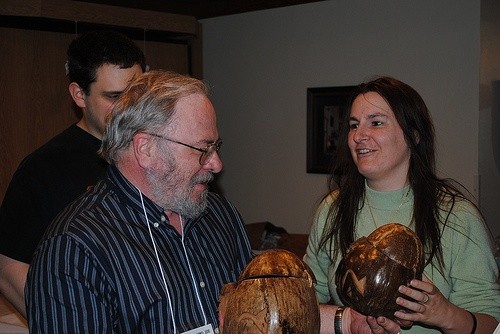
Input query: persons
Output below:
<box><xmin>302</xmin><ymin>76</ymin><xmax>500</xmax><ymax>334</ymax></box>
<box><xmin>23</xmin><ymin>66</ymin><xmax>417</xmax><ymax>334</ymax></box>
<box><xmin>0</xmin><ymin>28</ymin><xmax>147</xmax><ymax>320</ymax></box>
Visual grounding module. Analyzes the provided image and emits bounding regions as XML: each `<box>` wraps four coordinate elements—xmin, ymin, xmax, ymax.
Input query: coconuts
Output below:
<box><xmin>336</xmin><ymin>223</ymin><xmax>422</xmax><ymax>321</ymax></box>
<box><xmin>219</xmin><ymin>248</ymin><xmax>320</xmax><ymax>334</ymax></box>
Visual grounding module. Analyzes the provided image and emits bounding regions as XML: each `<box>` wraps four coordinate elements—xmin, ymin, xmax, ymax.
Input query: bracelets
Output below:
<box><xmin>334</xmin><ymin>305</ymin><xmax>347</xmax><ymax>334</ymax></box>
<box><xmin>467</xmin><ymin>310</ymin><xmax>477</xmax><ymax>334</ymax></box>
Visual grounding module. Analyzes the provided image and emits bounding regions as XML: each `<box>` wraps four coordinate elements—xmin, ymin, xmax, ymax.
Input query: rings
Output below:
<box><xmin>419</xmin><ymin>293</ymin><xmax>429</xmax><ymax>305</ymax></box>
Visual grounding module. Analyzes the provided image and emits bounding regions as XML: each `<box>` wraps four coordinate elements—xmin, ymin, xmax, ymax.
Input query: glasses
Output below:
<box><xmin>131</xmin><ymin>130</ymin><xmax>223</xmax><ymax>166</ymax></box>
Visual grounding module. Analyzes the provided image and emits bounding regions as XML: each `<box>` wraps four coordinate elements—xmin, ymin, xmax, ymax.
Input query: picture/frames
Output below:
<box><xmin>307</xmin><ymin>86</ymin><xmax>357</xmax><ymax>176</ymax></box>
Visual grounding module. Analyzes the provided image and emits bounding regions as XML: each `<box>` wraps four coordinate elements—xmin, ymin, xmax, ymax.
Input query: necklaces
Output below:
<box><xmin>365</xmin><ymin>186</ymin><xmax>413</xmax><ymax>233</ymax></box>
